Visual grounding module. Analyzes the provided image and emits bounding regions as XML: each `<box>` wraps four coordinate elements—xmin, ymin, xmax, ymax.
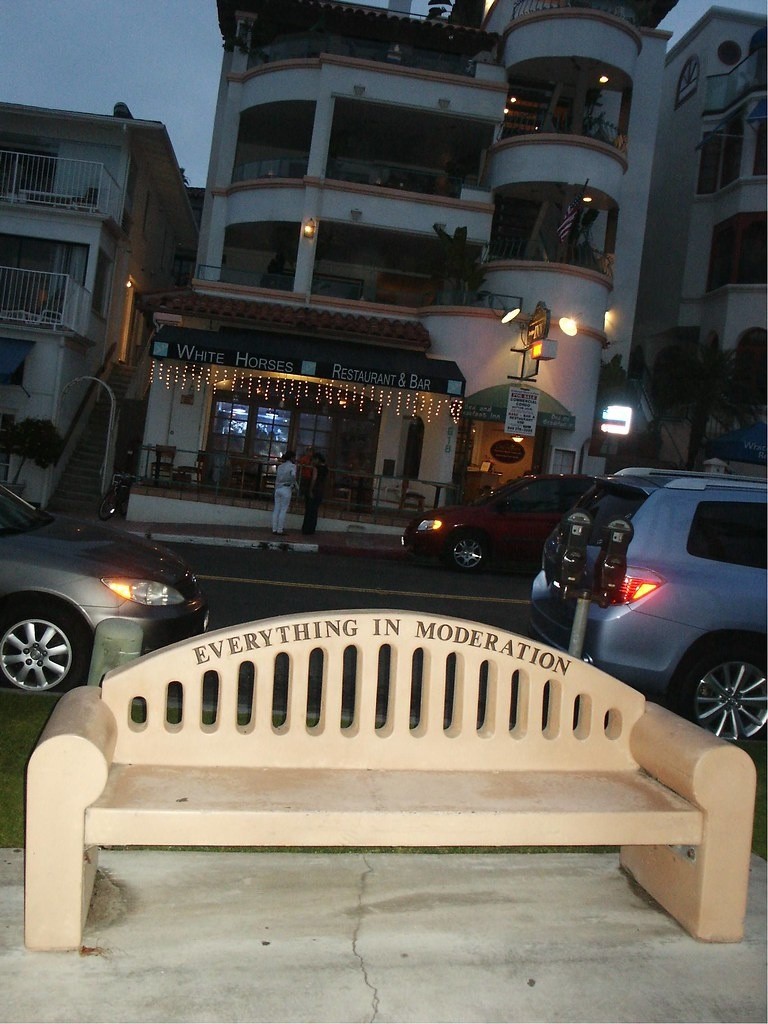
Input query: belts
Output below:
<box><xmin>284</xmin><ymin>484</ymin><xmax>290</xmax><ymax>486</ymax></box>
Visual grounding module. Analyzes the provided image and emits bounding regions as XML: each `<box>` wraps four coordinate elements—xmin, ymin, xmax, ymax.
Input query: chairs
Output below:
<box><xmin>222</xmin><ymin>466</ymin><xmax>246</xmax><ymax>496</ymax></box>
<box><xmin>0</xmin><ymin>310</ymin><xmax>63</xmax><ymax>325</ymax></box>
<box><xmin>330</xmin><ymin>487</ymin><xmax>351</xmax><ymax>513</ymax></box>
<box><xmin>400</xmin><ymin>479</ymin><xmax>425</xmax><ymax>514</ymax></box>
<box><xmin>151</xmin><ymin>444</ymin><xmax>176</xmax><ymax>479</ymax></box>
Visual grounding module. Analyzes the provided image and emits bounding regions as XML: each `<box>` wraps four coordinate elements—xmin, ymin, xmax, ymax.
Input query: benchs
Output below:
<box><xmin>20</xmin><ymin>189</ymin><xmax>85</xmax><ymax>207</ymax></box>
<box><xmin>26</xmin><ymin>610</ymin><xmax>756</xmax><ymax>952</ymax></box>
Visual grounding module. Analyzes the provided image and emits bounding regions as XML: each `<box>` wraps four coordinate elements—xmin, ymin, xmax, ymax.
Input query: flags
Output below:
<box><xmin>557</xmin><ymin>190</ymin><xmax>585</xmax><ymax>243</ymax></box>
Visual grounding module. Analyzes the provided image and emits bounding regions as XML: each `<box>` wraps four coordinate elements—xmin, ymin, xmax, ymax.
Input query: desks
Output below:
<box><xmin>178</xmin><ymin>466</ymin><xmax>202</xmax><ymax>483</ymax></box>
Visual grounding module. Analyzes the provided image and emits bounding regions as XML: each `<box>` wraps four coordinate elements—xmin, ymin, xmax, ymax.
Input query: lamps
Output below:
<box><xmin>304</xmin><ymin>218</ymin><xmax>316</xmax><ymax>237</ymax></box>
<box><xmin>479</xmin><ymin>291</ymin><xmax>524</xmax><ymax>323</ymax></box>
<box><xmin>558</xmin><ymin>312</ymin><xmax>585</xmax><ymax>336</ymax></box>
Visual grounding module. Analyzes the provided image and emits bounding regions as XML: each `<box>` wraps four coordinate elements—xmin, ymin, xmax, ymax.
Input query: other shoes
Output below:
<box><xmin>274</xmin><ymin>532</ymin><xmax>277</xmax><ymax>535</ymax></box>
<box><xmin>300</xmin><ymin>531</ymin><xmax>314</xmax><ymax>535</ymax></box>
<box><xmin>277</xmin><ymin>531</ymin><xmax>288</xmax><ymax>535</ymax></box>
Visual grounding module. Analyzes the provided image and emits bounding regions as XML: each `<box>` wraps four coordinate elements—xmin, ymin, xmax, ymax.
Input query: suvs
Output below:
<box><xmin>527</xmin><ymin>467</ymin><xmax>767</xmax><ymax>741</ymax></box>
<box><xmin>402</xmin><ymin>471</ymin><xmax>615</xmax><ymax>575</ymax></box>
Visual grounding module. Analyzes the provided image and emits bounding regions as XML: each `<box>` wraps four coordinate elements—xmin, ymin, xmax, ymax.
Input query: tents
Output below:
<box><xmin>706</xmin><ymin>421</ymin><xmax>767</xmax><ymax>464</ymax></box>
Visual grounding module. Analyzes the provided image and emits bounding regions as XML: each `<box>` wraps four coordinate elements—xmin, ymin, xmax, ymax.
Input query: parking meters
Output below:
<box><xmin>550</xmin><ymin>513</ymin><xmax>633</xmax><ymax>658</ymax></box>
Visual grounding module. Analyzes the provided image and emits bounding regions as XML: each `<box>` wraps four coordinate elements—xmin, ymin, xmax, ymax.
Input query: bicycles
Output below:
<box><xmin>99</xmin><ymin>466</ymin><xmax>131</xmax><ymax>520</ymax></box>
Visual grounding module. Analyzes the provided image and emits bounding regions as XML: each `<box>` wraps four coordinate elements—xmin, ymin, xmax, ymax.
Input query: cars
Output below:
<box><xmin>0</xmin><ymin>483</ymin><xmax>212</xmax><ymax>696</ymax></box>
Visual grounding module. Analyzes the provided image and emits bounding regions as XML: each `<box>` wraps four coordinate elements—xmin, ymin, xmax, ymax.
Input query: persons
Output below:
<box><xmin>216</xmin><ymin>430</ymin><xmax>276</xmax><ymax>455</ymax></box>
<box><xmin>272</xmin><ymin>451</ymin><xmax>296</xmax><ymax>535</ymax></box>
<box><xmin>298</xmin><ymin>446</ymin><xmax>315</xmax><ymax>496</ymax></box>
<box><xmin>302</xmin><ymin>452</ymin><xmax>328</xmax><ymax>535</ymax></box>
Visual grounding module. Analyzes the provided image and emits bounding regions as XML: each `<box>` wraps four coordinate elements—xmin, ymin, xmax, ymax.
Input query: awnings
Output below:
<box><xmin>149</xmin><ymin>325</ymin><xmax>467</xmax><ymax>398</ymax></box>
<box><xmin>0</xmin><ymin>337</ymin><xmax>37</xmax><ymax>374</ymax></box>
<box><xmin>462</xmin><ymin>383</ymin><xmax>576</xmax><ymax>432</ymax></box>
